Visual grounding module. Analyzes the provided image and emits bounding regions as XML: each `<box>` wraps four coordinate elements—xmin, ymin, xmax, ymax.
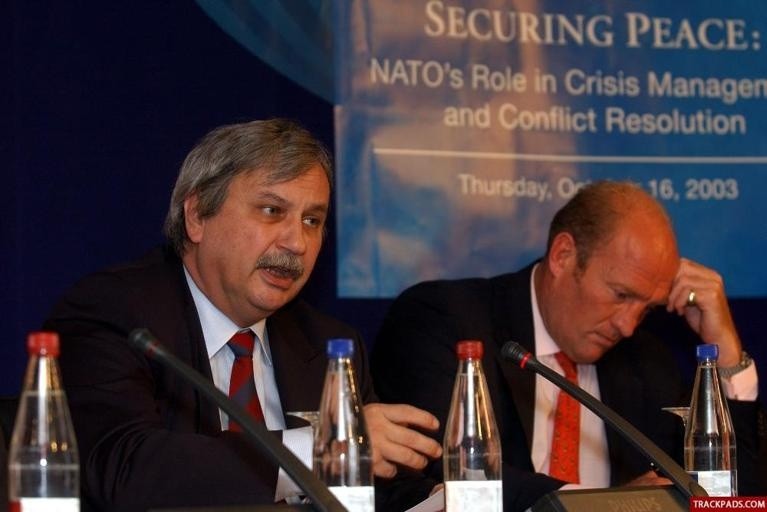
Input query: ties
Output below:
<box><xmin>227</xmin><ymin>332</ymin><xmax>268</xmax><ymax>431</ymax></box>
<box><xmin>547</xmin><ymin>351</ymin><xmax>581</xmax><ymax>486</ymax></box>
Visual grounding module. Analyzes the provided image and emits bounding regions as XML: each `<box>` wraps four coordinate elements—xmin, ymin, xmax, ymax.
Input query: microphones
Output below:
<box><xmin>499</xmin><ymin>339</ymin><xmax>709</xmax><ymax>501</ymax></box>
<box><xmin>125</xmin><ymin>326</ymin><xmax>350</xmax><ymax>512</ymax></box>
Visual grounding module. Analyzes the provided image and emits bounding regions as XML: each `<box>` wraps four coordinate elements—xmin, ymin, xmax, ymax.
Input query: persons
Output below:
<box><xmin>40</xmin><ymin>117</ymin><xmax>767</xmax><ymax>512</ymax></box>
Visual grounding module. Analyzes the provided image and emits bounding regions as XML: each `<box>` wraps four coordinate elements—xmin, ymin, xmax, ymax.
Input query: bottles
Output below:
<box><xmin>679</xmin><ymin>342</ymin><xmax>736</xmax><ymax>499</ymax></box>
<box><xmin>312</xmin><ymin>339</ymin><xmax>376</xmax><ymax>512</ymax></box>
<box><xmin>442</xmin><ymin>342</ymin><xmax>506</xmax><ymax>512</ymax></box>
<box><xmin>7</xmin><ymin>332</ymin><xmax>79</xmax><ymax>512</ymax></box>
